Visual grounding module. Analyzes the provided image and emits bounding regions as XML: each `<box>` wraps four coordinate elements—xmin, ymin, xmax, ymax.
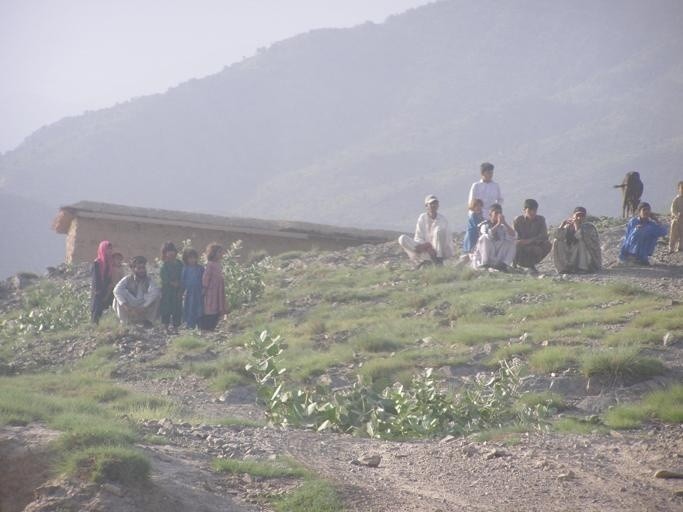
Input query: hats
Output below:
<box><xmin>424</xmin><ymin>195</ymin><xmax>437</xmax><ymax>205</ymax></box>
<box><xmin>572</xmin><ymin>207</ymin><xmax>585</xmax><ymax>215</ymax></box>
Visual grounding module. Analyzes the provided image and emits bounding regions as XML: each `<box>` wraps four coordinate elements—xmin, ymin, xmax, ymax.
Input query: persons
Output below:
<box><xmin>201</xmin><ymin>244</ymin><xmax>228</xmax><ymax>333</ymax></box>
<box><xmin>463</xmin><ymin>199</ymin><xmax>483</xmax><ymax>254</ymax></box>
<box><xmin>617</xmin><ymin>201</ymin><xmax>666</xmax><ymax>267</ymax></box>
<box><xmin>670</xmin><ymin>182</ymin><xmax>683</xmax><ymax>255</ymax></box>
<box><xmin>550</xmin><ymin>206</ymin><xmax>603</xmax><ymax>275</ymax></box>
<box><xmin>469</xmin><ymin>203</ymin><xmax>518</xmax><ymax>273</ymax></box>
<box><xmin>398</xmin><ymin>194</ymin><xmax>454</xmax><ymax>269</ymax></box>
<box><xmin>467</xmin><ymin>161</ymin><xmax>504</xmax><ymax>220</ymax></box>
<box><xmin>181</xmin><ymin>248</ymin><xmax>204</xmax><ymax>331</ymax></box>
<box><xmin>110</xmin><ymin>251</ymin><xmax>125</xmax><ymax>287</ymax></box>
<box><xmin>111</xmin><ymin>256</ymin><xmax>161</xmax><ymax>329</ymax></box>
<box><xmin>512</xmin><ymin>199</ymin><xmax>552</xmax><ymax>273</ymax></box>
<box><xmin>90</xmin><ymin>241</ymin><xmax>113</xmax><ymax>323</ymax></box>
<box><xmin>160</xmin><ymin>241</ymin><xmax>184</xmax><ymax>334</ymax></box>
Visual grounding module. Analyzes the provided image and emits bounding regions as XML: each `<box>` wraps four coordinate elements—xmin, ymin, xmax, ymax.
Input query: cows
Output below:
<box><xmin>613</xmin><ymin>171</ymin><xmax>643</xmax><ymax>218</ymax></box>
